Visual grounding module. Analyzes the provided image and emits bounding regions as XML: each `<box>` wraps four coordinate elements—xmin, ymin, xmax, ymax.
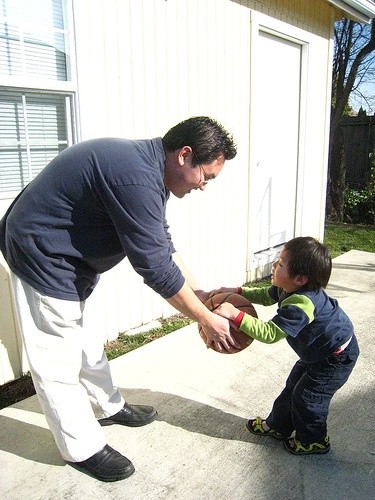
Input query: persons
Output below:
<box><xmin>0</xmin><ymin>117</ymin><xmax>237</xmax><ymax>483</ymax></box>
<box><xmin>207</xmin><ymin>236</ymin><xmax>359</xmax><ymax>456</ymax></box>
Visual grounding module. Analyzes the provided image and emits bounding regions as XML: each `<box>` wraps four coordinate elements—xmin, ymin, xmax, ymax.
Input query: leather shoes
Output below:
<box><xmin>69</xmin><ymin>443</ymin><xmax>135</xmax><ymax>482</ymax></box>
<box><xmin>97</xmin><ymin>402</ymin><xmax>157</xmax><ymax>426</ymax></box>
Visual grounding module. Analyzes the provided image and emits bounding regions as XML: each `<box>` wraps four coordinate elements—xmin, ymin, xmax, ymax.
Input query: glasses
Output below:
<box><xmin>184</xmin><ymin>144</ymin><xmax>212</xmax><ymax>185</ymax></box>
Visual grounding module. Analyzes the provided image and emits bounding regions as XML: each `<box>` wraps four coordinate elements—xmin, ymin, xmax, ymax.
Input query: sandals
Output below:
<box><xmin>246</xmin><ymin>417</ymin><xmax>287</xmax><ymax>440</ymax></box>
<box><xmin>284</xmin><ymin>433</ymin><xmax>330</xmax><ymax>455</ymax></box>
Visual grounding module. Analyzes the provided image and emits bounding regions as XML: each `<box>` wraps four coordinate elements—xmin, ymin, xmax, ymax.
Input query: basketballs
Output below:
<box><xmin>198</xmin><ymin>291</ymin><xmax>259</xmax><ymax>357</ymax></box>
